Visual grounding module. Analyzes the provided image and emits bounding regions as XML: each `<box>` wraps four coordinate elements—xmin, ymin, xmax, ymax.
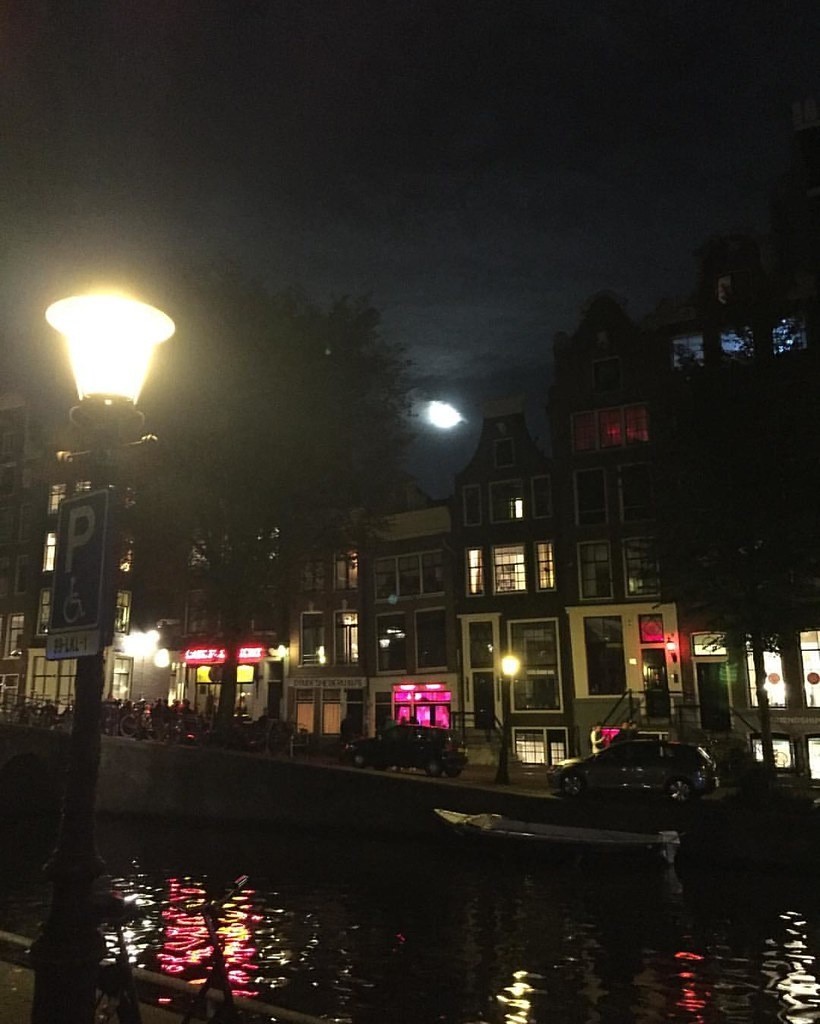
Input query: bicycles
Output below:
<box><xmin>120</xmin><ymin>707</ymin><xmax>157</xmax><ymax>737</ymax></box>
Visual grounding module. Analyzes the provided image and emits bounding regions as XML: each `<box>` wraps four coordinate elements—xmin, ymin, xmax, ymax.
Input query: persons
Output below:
<box><xmin>0</xmin><ymin>686</ymin><xmax>317</xmax><ymax>756</ymax></box>
<box><xmin>588</xmin><ymin>720</ymin><xmax>642</xmax><ymax>760</ymax></box>
<box><xmin>383</xmin><ymin>711</ymin><xmax>421</xmax><ymax>777</ymax></box>
<box><xmin>341</xmin><ymin>712</ymin><xmax>353</xmax><ymax>750</ymax></box>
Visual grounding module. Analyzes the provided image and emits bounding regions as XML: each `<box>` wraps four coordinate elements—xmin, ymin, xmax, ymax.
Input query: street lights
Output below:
<box><xmin>31</xmin><ymin>287</ymin><xmax>175</xmax><ymax>1024</ymax></box>
<box><xmin>492</xmin><ymin>655</ymin><xmax>521</xmax><ymax>784</ymax></box>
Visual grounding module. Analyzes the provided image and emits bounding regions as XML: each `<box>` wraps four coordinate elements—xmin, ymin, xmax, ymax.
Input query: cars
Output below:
<box><xmin>558</xmin><ymin>740</ymin><xmax>720</xmax><ymax>805</ymax></box>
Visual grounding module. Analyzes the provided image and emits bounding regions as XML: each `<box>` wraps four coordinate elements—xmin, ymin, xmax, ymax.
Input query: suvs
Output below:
<box><xmin>337</xmin><ymin>724</ymin><xmax>469</xmax><ymax>778</ymax></box>
